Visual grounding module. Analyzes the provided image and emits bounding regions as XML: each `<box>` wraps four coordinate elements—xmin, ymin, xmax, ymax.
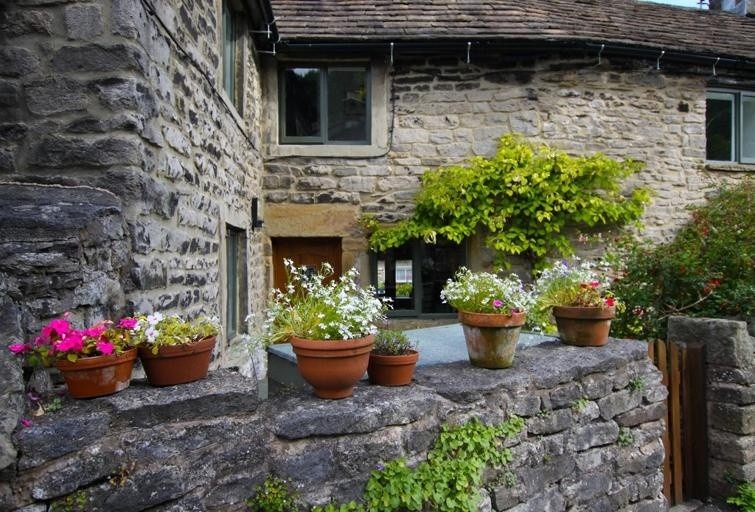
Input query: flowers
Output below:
<box><xmin>228</xmin><ymin>256</ymin><xmax>395</xmax><ymax>353</ymax></box>
<box><xmin>533</xmin><ymin>271</ymin><xmax>616</xmax><ymax>315</ymax></box>
<box><xmin>438</xmin><ymin>264</ymin><xmax>537</xmax><ymax>316</ymax></box>
<box><xmin>7</xmin><ymin>313</ymin><xmax>141</xmax><ymax>434</ymax></box>
<box><xmin>373</xmin><ymin>328</ymin><xmax>418</xmax><ymax>355</ymax></box>
<box><xmin>127</xmin><ymin>308</ymin><xmax>219</xmax><ymax>355</ymax></box>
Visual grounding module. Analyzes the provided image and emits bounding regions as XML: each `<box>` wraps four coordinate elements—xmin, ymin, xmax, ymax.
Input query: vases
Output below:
<box><xmin>136</xmin><ymin>336</ymin><xmax>216</xmax><ymax>387</ymax></box>
<box><xmin>457</xmin><ymin>309</ymin><xmax>527</xmax><ymax>368</ymax></box>
<box><xmin>367</xmin><ymin>349</ymin><xmax>419</xmax><ymax>386</ymax></box>
<box><xmin>290</xmin><ymin>334</ymin><xmax>374</xmax><ymax>399</ymax></box>
<box><xmin>50</xmin><ymin>349</ymin><xmax>137</xmax><ymax>399</ymax></box>
<box><xmin>551</xmin><ymin>306</ymin><xmax>615</xmax><ymax>347</ymax></box>
